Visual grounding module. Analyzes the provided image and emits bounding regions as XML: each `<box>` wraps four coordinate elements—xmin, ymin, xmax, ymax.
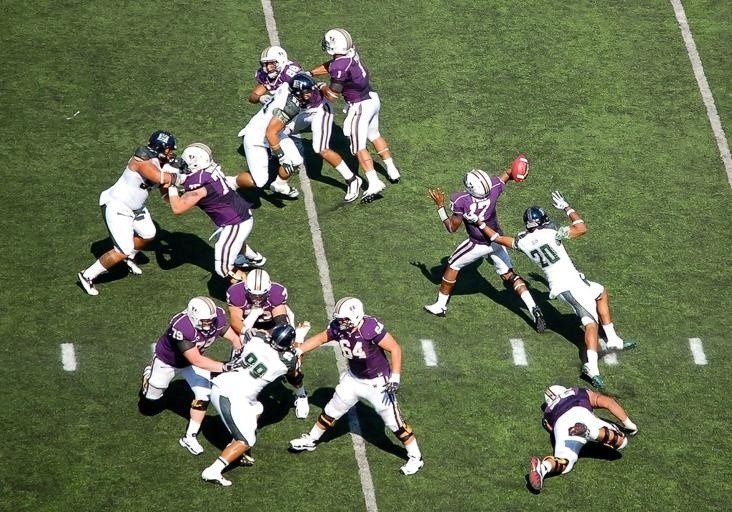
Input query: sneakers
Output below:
<box><xmin>179</xmin><ymin>433</ymin><xmax>203</xmax><ymax>455</ymax></box>
<box><xmin>361</xmin><ymin>181</ymin><xmax>386</xmax><ymax>203</ymax></box>
<box><xmin>201</xmin><ymin>468</ymin><xmax>233</xmax><ymax>487</ymax></box>
<box><xmin>273</xmin><ymin>179</ymin><xmax>300</xmax><ymax>198</ymax></box>
<box><xmin>581</xmin><ymin>363</ymin><xmax>605</xmax><ymax>390</ymax></box>
<box><xmin>533</xmin><ymin>306</ymin><xmax>546</xmax><ymax>333</ymax></box>
<box><xmin>123</xmin><ymin>259</ymin><xmax>143</xmax><ymax>275</ymax></box>
<box><xmin>294</xmin><ymin>397</ymin><xmax>309</xmax><ymax>419</ymax></box>
<box><xmin>247</xmin><ymin>252</ymin><xmax>266</xmax><ymax>267</ymax></box>
<box><xmin>399</xmin><ymin>454</ymin><xmax>424</xmax><ymax>475</ymax></box>
<box><xmin>606</xmin><ymin>339</ymin><xmax>636</xmax><ymax>351</ymax></box>
<box><xmin>344</xmin><ymin>175</ymin><xmax>362</xmax><ymax>202</ymax></box>
<box><xmin>382</xmin><ymin>159</ymin><xmax>400</xmax><ymax>181</ymax></box>
<box><xmin>530</xmin><ymin>456</ymin><xmax>544</xmax><ymax>490</ymax></box>
<box><xmin>289</xmin><ymin>433</ymin><xmax>316</xmax><ymax>452</ymax></box>
<box><xmin>143</xmin><ymin>366</ymin><xmax>151</xmax><ymax>377</ymax></box>
<box><xmin>569</xmin><ymin>423</ymin><xmax>590</xmax><ymax>437</ymax></box>
<box><xmin>225</xmin><ymin>175</ymin><xmax>239</xmax><ymax>192</ymax></box>
<box><xmin>424</xmin><ymin>302</ymin><xmax>447</xmax><ymax>317</ymax></box>
<box><xmin>226</xmin><ymin>443</ymin><xmax>254</xmax><ymax>466</ymax></box>
<box><xmin>77</xmin><ymin>270</ymin><xmax>99</xmax><ymax>296</ymax></box>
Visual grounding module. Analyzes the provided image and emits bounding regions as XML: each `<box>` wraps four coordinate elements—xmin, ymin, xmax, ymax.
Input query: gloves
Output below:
<box><xmin>623</xmin><ymin>417</ymin><xmax>638</xmax><ymax>436</ymax></box>
<box><xmin>241</xmin><ymin>327</ymin><xmax>257</xmax><ymax>344</ymax></box>
<box><xmin>259</xmin><ymin>95</ymin><xmax>272</xmax><ymax>104</ymax></box>
<box><xmin>170</xmin><ymin>174</ymin><xmax>187</xmax><ymax>186</ymax></box>
<box><xmin>312</xmin><ymin>82</ymin><xmax>326</xmax><ymax>90</ymax></box>
<box><xmin>222</xmin><ymin>359</ymin><xmax>241</xmax><ymax>372</ymax></box>
<box><xmin>231</xmin><ymin>347</ymin><xmax>244</xmax><ymax>360</ymax></box>
<box><xmin>552</xmin><ymin>190</ymin><xmax>569</xmax><ymax>210</ymax></box>
<box><xmin>381</xmin><ymin>383</ymin><xmax>400</xmax><ymax>401</ymax></box>
<box><xmin>280</xmin><ymin>157</ymin><xmax>294</xmax><ymax>175</ymax></box>
<box><xmin>463</xmin><ymin>212</ymin><xmax>478</xmax><ymax>223</ymax></box>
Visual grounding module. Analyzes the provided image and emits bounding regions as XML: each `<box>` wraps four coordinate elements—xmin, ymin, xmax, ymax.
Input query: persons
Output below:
<box><xmin>76</xmin><ymin>129</ymin><xmax>191</xmax><ymax>295</ymax></box>
<box><xmin>141</xmin><ymin>269</ymin><xmax>310</xmax><ymax>485</ymax></box>
<box><xmin>289</xmin><ymin>296</ymin><xmax>424</xmax><ymax>477</ymax></box>
<box><xmin>528</xmin><ymin>382</ymin><xmax>639</xmax><ymax>491</ymax></box>
<box><xmin>460</xmin><ymin>190</ymin><xmax>636</xmax><ymax>388</ymax></box>
<box><xmin>165</xmin><ymin>141</ymin><xmax>268</xmax><ymax>286</ymax></box>
<box><xmin>420</xmin><ymin>153</ymin><xmax>548</xmax><ymax>333</ymax></box>
<box><xmin>223</xmin><ymin>27</ymin><xmax>401</xmax><ymax>207</ymax></box>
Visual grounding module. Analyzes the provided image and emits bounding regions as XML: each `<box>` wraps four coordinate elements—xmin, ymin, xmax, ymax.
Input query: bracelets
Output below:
<box><xmin>479</xmin><ymin>222</ymin><xmax>487</xmax><ymax>230</ymax></box>
<box><xmin>168</xmin><ymin>186</ymin><xmax>179</xmax><ymax>198</ymax></box>
<box><xmin>436</xmin><ymin>206</ymin><xmax>449</xmax><ymax>222</ymax></box>
<box><xmin>566</xmin><ymin>209</ymin><xmax>576</xmax><ymax>217</ymax></box>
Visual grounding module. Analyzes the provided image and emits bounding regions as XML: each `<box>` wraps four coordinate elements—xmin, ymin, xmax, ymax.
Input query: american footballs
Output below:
<box><xmin>512</xmin><ymin>154</ymin><xmax>529</xmax><ymax>184</ymax></box>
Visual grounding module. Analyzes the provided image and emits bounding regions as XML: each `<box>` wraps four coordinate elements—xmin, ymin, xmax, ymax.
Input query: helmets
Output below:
<box><xmin>147</xmin><ymin>130</ymin><xmax>177</xmax><ymax>161</ymax></box>
<box><xmin>332</xmin><ymin>297</ymin><xmax>364</xmax><ymax>333</ymax></box>
<box><xmin>289</xmin><ymin>74</ymin><xmax>318</xmax><ymax>99</ymax></box>
<box><xmin>322</xmin><ymin>28</ymin><xmax>353</xmax><ymax>56</ymax></box>
<box><xmin>179</xmin><ymin>143</ymin><xmax>213</xmax><ymax>176</ymax></box>
<box><xmin>463</xmin><ymin>169</ymin><xmax>492</xmax><ymax>199</ymax></box>
<box><xmin>522</xmin><ymin>206</ymin><xmax>551</xmax><ymax>231</ymax></box>
<box><xmin>245</xmin><ymin>269</ymin><xmax>271</xmax><ymax>306</ymax></box>
<box><xmin>269</xmin><ymin>324</ymin><xmax>296</xmax><ymax>351</ymax></box>
<box><xmin>545</xmin><ymin>385</ymin><xmax>567</xmax><ymax>404</ymax></box>
<box><xmin>187</xmin><ymin>296</ymin><xmax>218</xmax><ymax>337</ymax></box>
<box><xmin>260</xmin><ymin>46</ymin><xmax>288</xmax><ymax>79</ymax></box>
